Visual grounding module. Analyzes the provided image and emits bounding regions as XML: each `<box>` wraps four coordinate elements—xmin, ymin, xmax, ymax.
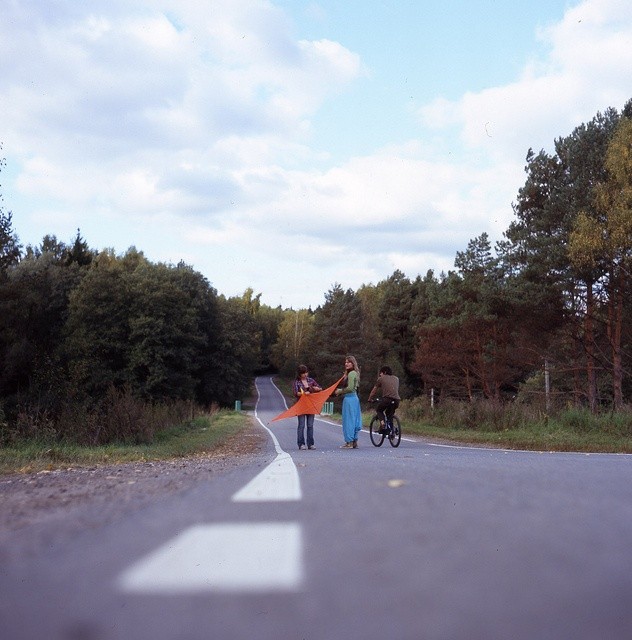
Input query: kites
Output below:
<box><xmin>270</xmin><ymin>374</ymin><xmax>347</xmax><ymax>422</ymax></box>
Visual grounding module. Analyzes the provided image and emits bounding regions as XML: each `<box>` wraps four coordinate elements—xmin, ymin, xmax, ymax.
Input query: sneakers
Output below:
<box><xmin>380</xmin><ymin>420</ymin><xmax>385</xmax><ymax>428</ymax></box>
<box><xmin>311</xmin><ymin>445</ymin><xmax>316</xmax><ymax>450</ymax></box>
<box><xmin>339</xmin><ymin>442</ymin><xmax>354</xmax><ymax>449</ymax></box>
<box><xmin>353</xmin><ymin>441</ymin><xmax>359</xmax><ymax>449</ymax></box>
<box><xmin>300</xmin><ymin>444</ymin><xmax>308</xmax><ymax>450</ymax></box>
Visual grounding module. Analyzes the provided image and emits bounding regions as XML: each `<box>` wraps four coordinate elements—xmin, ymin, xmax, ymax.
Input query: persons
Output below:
<box><xmin>368</xmin><ymin>366</ymin><xmax>401</xmax><ymax>439</ymax></box>
<box><xmin>335</xmin><ymin>356</ymin><xmax>362</xmax><ymax>448</ymax></box>
<box><xmin>292</xmin><ymin>364</ymin><xmax>322</xmax><ymax>450</ymax></box>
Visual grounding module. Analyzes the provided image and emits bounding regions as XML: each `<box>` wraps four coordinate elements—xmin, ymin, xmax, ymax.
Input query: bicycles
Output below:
<box><xmin>369</xmin><ymin>398</ymin><xmax>401</xmax><ymax>448</ymax></box>
